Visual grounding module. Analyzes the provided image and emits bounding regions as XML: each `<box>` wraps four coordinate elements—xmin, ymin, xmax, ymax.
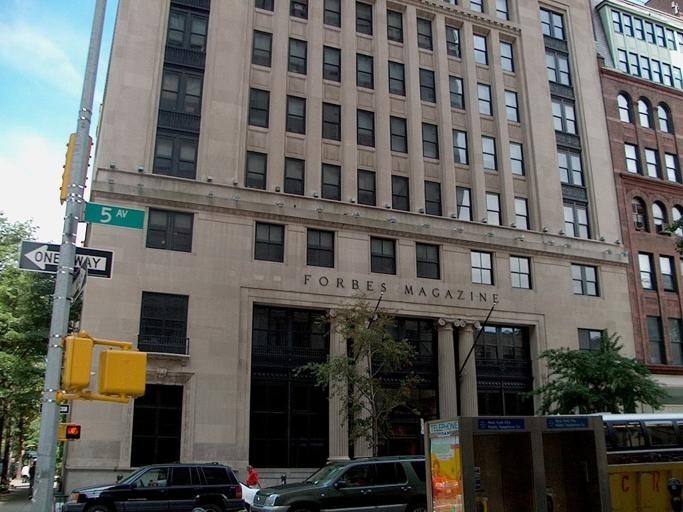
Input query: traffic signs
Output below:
<box><xmin>81</xmin><ymin>201</ymin><xmax>147</xmax><ymax>230</ymax></box>
<box><xmin>39</xmin><ymin>403</ymin><xmax>70</xmax><ymax>414</ymax></box>
<box><xmin>17</xmin><ymin>239</ymin><xmax>115</xmax><ymax>282</ymax></box>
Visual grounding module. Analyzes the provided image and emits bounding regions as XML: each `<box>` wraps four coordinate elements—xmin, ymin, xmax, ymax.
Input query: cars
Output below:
<box><xmin>238</xmin><ymin>482</ymin><xmax>260</xmax><ymax>511</ymax></box>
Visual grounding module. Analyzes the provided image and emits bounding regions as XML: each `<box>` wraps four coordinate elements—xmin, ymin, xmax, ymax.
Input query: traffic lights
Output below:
<box><xmin>65</xmin><ymin>424</ymin><xmax>81</xmax><ymax>441</ymax></box>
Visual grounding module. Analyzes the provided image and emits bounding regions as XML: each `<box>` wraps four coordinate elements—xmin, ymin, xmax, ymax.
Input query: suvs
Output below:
<box><xmin>252</xmin><ymin>457</ymin><xmax>427</xmax><ymax>512</ymax></box>
<box><xmin>64</xmin><ymin>462</ymin><xmax>246</xmax><ymax>512</ymax></box>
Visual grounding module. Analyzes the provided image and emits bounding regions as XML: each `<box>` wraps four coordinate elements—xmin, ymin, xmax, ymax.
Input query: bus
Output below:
<box><xmin>602</xmin><ymin>414</ymin><xmax>682</xmax><ymax>512</ymax></box>
